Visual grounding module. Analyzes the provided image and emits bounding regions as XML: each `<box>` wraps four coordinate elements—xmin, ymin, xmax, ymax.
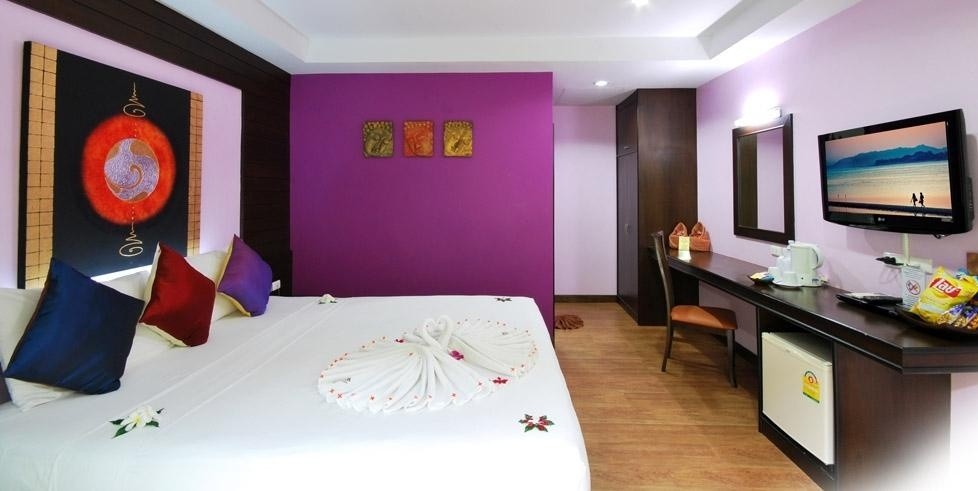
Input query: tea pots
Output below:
<box><xmin>788</xmin><ymin>240</ymin><xmax>823</xmax><ymax>285</ymax></box>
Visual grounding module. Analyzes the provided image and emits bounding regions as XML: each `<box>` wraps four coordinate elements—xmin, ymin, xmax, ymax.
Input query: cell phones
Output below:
<box><xmin>863</xmin><ymin>295</ymin><xmax>902</xmax><ymax>304</ymax></box>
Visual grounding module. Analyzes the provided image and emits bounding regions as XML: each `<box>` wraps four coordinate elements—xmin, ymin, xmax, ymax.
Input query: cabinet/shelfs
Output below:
<box><xmin>615</xmin><ymin>89</ymin><xmax>697</xmax><ymax>326</ymax></box>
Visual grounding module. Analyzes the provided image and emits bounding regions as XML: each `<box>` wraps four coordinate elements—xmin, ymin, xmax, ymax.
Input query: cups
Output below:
<box><xmin>678</xmin><ymin>236</ymin><xmax>690</xmax><ymax>251</ymax></box>
<box><xmin>768</xmin><ymin>267</ymin><xmax>783</xmax><ymax>282</ymax></box>
<box><xmin>678</xmin><ymin>251</ymin><xmax>691</xmax><ymax>262</ymax></box>
<box><xmin>783</xmin><ymin>270</ymin><xmax>798</xmax><ymax>288</ymax></box>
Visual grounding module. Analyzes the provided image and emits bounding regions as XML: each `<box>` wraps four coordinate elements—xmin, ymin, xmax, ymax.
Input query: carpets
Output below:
<box><xmin>554</xmin><ymin>315</ymin><xmax>584</xmax><ymax>332</ymax></box>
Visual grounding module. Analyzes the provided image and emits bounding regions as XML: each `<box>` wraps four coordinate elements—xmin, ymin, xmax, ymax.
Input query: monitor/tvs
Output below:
<box><xmin>817</xmin><ymin>108</ymin><xmax>974</xmax><ymax>236</ymax></box>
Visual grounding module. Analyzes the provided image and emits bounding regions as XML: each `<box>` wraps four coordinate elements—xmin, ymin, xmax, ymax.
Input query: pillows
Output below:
<box><xmin>148</xmin><ymin>250</ymin><xmax>239</xmax><ymax>346</ymax></box>
<box><xmin>0</xmin><ymin>289</ymin><xmax>81</xmax><ymax>416</ymax></box>
<box><xmin>139</xmin><ymin>242</ymin><xmax>218</xmax><ymax>347</ymax></box>
<box><xmin>2</xmin><ymin>258</ymin><xmax>146</xmax><ymax>394</ymax></box>
<box><xmin>0</xmin><ymin>258</ymin><xmax>152</xmax><ymax>411</ymax></box>
<box><xmin>217</xmin><ymin>234</ymin><xmax>272</xmax><ymax>317</ymax></box>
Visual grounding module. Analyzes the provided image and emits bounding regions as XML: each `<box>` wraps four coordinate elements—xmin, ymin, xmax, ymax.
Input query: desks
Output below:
<box><xmin>667</xmin><ymin>254</ymin><xmax>978</xmax><ymax>491</ymax></box>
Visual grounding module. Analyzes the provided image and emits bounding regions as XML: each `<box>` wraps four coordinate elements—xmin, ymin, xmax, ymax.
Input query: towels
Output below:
<box><xmin>315</xmin><ymin>313</ymin><xmax>538</xmax><ymax>418</ymax></box>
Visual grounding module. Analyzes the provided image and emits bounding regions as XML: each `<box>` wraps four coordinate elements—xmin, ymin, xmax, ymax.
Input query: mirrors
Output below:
<box><xmin>732</xmin><ymin>114</ymin><xmax>794</xmax><ymax>244</ymax></box>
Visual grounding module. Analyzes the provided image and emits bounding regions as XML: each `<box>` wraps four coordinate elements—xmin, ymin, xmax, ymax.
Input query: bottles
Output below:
<box><xmin>776</xmin><ymin>247</ymin><xmax>791</xmax><ymax>271</ymax></box>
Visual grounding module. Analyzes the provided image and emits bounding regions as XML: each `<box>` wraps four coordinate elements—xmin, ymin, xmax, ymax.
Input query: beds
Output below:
<box><xmin>0</xmin><ymin>297</ymin><xmax>591</xmax><ymax>491</ymax></box>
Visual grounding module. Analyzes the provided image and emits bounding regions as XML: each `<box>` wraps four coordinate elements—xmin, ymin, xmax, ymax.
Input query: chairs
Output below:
<box><xmin>652</xmin><ymin>230</ymin><xmax>736</xmax><ymax>387</ymax></box>
